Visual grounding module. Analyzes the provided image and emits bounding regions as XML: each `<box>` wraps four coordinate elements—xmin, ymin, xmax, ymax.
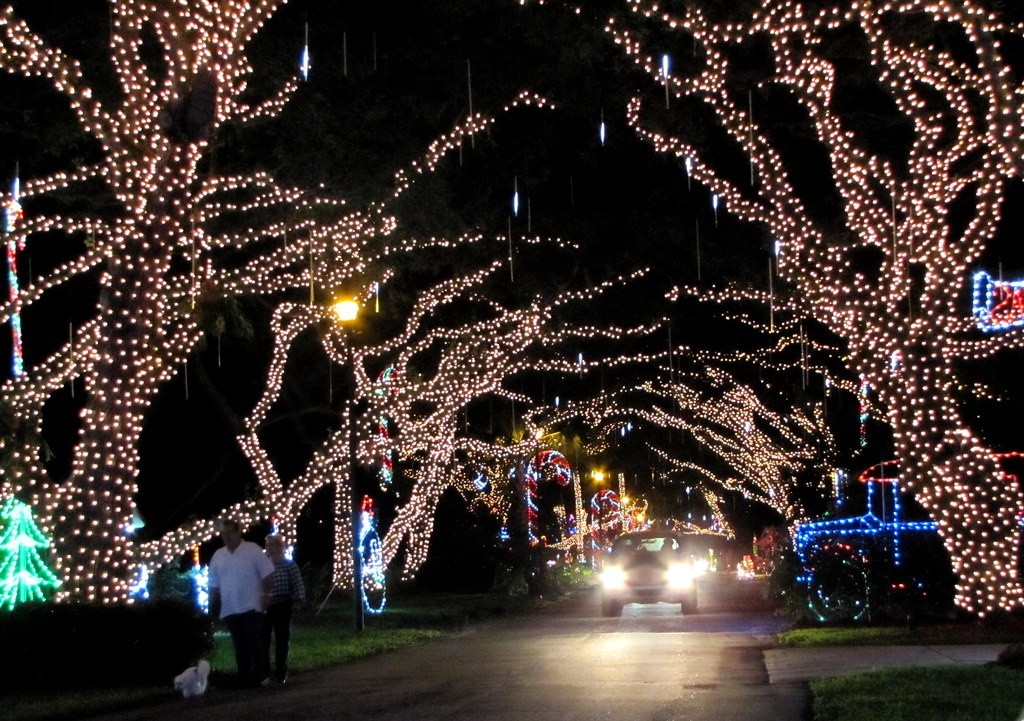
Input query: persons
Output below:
<box><xmin>257</xmin><ymin>536</ymin><xmax>306</xmax><ymax>686</ymax></box>
<box><xmin>209</xmin><ymin>520</ymin><xmax>275</xmax><ymax>691</ymax></box>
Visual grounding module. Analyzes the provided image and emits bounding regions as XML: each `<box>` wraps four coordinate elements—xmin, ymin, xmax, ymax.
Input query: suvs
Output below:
<box><xmin>600</xmin><ymin>530</ymin><xmax>699</xmax><ymax>617</ymax></box>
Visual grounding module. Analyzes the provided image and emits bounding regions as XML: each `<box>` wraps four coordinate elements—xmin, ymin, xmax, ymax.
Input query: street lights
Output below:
<box><xmin>593</xmin><ymin>467</ymin><xmax>605</xmax><ymax>566</ymax></box>
<box><xmin>331</xmin><ymin>294</ymin><xmax>365</xmax><ymax>631</ymax></box>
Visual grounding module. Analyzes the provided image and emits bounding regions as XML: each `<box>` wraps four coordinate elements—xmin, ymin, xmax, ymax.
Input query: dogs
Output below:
<box><xmin>173</xmin><ymin>659</ymin><xmax>210</xmax><ymax>698</ymax></box>
